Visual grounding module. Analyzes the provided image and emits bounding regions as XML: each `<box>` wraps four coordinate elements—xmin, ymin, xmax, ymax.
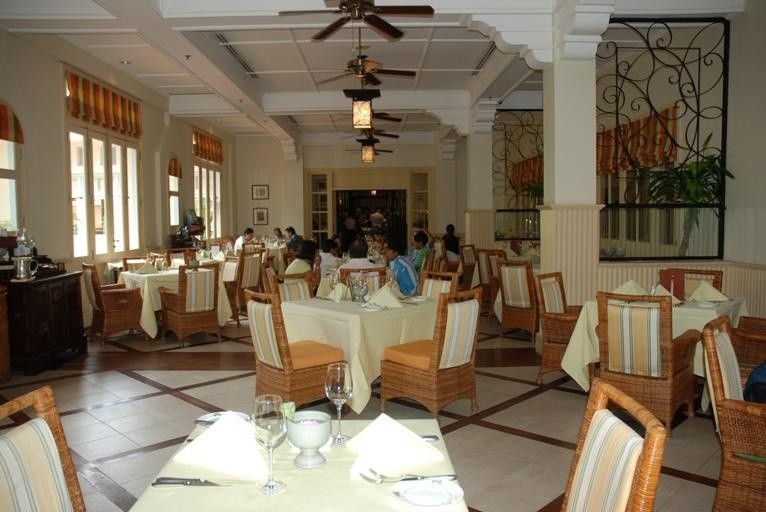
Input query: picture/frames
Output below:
<box><xmin>252</xmin><ymin>185</ymin><xmax>269</xmax><ymax>199</ymax></box>
<box><xmin>0</xmin><ymin>248</ymin><xmax>10</xmax><ymax>263</ymax></box>
<box><xmin>253</xmin><ymin>208</ymin><xmax>268</xmax><ymax>224</ymax></box>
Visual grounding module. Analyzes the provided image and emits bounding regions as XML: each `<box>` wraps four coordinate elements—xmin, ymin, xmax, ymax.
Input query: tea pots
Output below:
<box><xmin>14</xmin><ymin>256</ymin><xmax>39</xmax><ymax>280</ymax></box>
<box><xmin>16</xmin><ymin>224</ymin><xmax>33</xmax><ymax>248</ymax></box>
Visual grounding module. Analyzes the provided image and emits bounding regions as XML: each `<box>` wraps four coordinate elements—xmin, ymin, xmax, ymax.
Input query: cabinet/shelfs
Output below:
<box><xmin>8</xmin><ymin>271</ymin><xmax>82</xmax><ymax>376</ymax></box>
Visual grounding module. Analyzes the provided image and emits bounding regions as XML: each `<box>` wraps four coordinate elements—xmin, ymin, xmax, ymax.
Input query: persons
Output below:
<box><xmin>315</xmin><ymin>239</ymin><xmax>342</xmax><ymax>276</ymax></box>
<box><xmin>336</xmin><ymin>240</ymin><xmax>384</xmax><ymax>285</ymax></box>
<box><xmin>282</xmin><ymin>240</ymin><xmax>321</xmax><ymax>288</ymax></box>
<box><xmin>382</xmin><ymin>237</ymin><xmax>420</xmax><ymax>297</ymax></box>
<box><xmin>268</xmin><ymin>228</ymin><xmax>287</xmax><ymax>243</ymax></box>
<box><xmin>405</xmin><ymin>231</ymin><xmax>430</xmax><ymax>269</ymax></box>
<box><xmin>283</xmin><ymin>226</ymin><xmax>303</xmax><ymax>250</ymax></box>
<box><xmin>369</xmin><ymin>209</ymin><xmax>387</xmax><ymax>231</ymax></box>
<box><xmin>441</xmin><ymin>223</ymin><xmax>461</xmax><ymax>260</ymax></box>
<box><xmin>234</xmin><ymin>228</ymin><xmax>255</xmax><ymax>256</ymax></box>
<box><xmin>339</xmin><ymin>219</ymin><xmax>362</xmax><ymax>247</ymax></box>
<box><xmin>410</xmin><ymin>221</ymin><xmax>436</xmax><ymax>247</ymax></box>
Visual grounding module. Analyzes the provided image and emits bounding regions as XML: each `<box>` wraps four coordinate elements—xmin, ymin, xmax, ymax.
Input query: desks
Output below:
<box><xmin>153</xmin><ymin>253</ymin><xmax>226</xmax><ymax>271</ymax></box>
<box><xmin>172</xmin><ymin>252</ymin><xmax>240</xmax><ymax>283</ymax></box>
<box><xmin>242</xmin><ymin>245</ymin><xmax>288</xmax><ymax>280</ymax></box>
<box><xmin>130</xmin><ymin>412</ymin><xmax>468</xmax><ymax>512</ymax></box>
<box><xmin>241</xmin><ymin>298</ymin><xmax>444</xmax><ymax>414</ymax></box>
<box><xmin>560</xmin><ymin>297</ymin><xmax>752</xmax><ymax>413</ymax></box>
<box><xmin>118</xmin><ymin>267</ymin><xmax>233</xmax><ymax>340</ymax></box>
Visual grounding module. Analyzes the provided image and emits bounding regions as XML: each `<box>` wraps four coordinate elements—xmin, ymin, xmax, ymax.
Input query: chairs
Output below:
<box><xmin>122</xmin><ymin>257</ymin><xmax>156</xmax><ymax>272</ymax></box>
<box><xmin>420</xmin><ymin>271</ymin><xmax>458</xmax><ymax>296</ymax></box>
<box><xmin>703</xmin><ymin>315</ymin><xmax>766</xmax><ymax>512</ymax></box>
<box><xmin>340</xmin><ymin>268</ymin><xmax>386</xmax><ymax>299</ymax></box>
<box><xmin>82</xmin><ymin>263</ymin><xmax>142</xmax><ymax>344</ymax></box>
<box><xmin>538</xmin><ymin>378</ymin><xmax>666</xmax><ymax>512</ymax></box>
<box><xmin>493</xmin><ymin>259</ymin><xmax>536</xmax><ymax>341</ymax></box>
<box><xmin>157</xmin><ymin>263</ymin><xmax>221</xmax><ymax>346</ymax></box>
<box><xmin>262</xmin><ymin>263</ymin><xmax>314</xmax><ymax>301</ymax></box>
<box><xmin>0</xmin><ymin>387</ymin><xmax>86</xmax><ymax>512</ymax></box>
<box><xmin>477</xmin><ymin>249</ymin><xmax>507</xmax><ymax>321</ymax></box>
<box><xmin>460</xmin><ymin>245</ymin><xmax>477</xmax><ymax>289</ymax></box>
<box><xmin>245</xmin><ymin>289</ymin><xmax>344</xmax><ymax>410</ymax></box>
<box><xmin>165</xmin><ymin>247</ymin><xmax>196</xmax><ymax>267</ymax></box>
<box><xmin>381</xmin><ymin>287</ymin><xmax>484</xmax><ymax>426</ymax></box>
<box><xmin>591</xmin><ymin>292</ymin><xmax>695</xmax><ymax>438</ymax></box>
<box><xmin>719</xmin><ymin>316</ymin><xmax>766</xmax><ymax>364</ymax></box>
<box><xmin>658</xmin><ymin>269</ymin><xmax>723</xmax><ymax>299</ymax></box>
<box><xmin>535</xmin><ymin>273</ymin><xmax>598</xmax><ymax>401</ymax></box>
<box><xmin>433</xmin><ymin>238</ymin><xmax>458</xmax><ymax>280</ymax></box>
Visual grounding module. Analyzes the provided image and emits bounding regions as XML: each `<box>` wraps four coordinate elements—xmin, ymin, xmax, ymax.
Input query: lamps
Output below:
<box><xmin>357</xmin><ymin>134</ymin><xmax>379</xmax><ymax>162</ymax></box>
<box><xmin>343</xmin><ymin>83</ymin><xmax>379</xmax><ymax>128</ymax></box>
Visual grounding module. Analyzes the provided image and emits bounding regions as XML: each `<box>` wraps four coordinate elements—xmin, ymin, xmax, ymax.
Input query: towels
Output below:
<box><xmin>346</xmin><ymin>412</ymin><xmax>444</xmax><ymax>478</ymax></box>
<box><xmin>690</xmin><ymin>279</ymin><xmax>729</xmax><ymax>303</ymax></box>
<box><xmin>136</xmin><ymin>262</ymin><xmax>157</xmax><ymax>274</ymax></box>
<box><xmin>654</xmin><ymin>284</ymin><xmax>680</xmax><ymax>304</ymax></box>
<box><xmin>374</xmin><ymin>282</ymin><xmax>406</xmax><ymax>299</ymax></box>
<box><xmin>328</xmin><ymin>283</ymin><xmax>352</xmax><ymax>300</ymax></box>
<box><xmin>179</xmin><ymin>409</ymin><xmax>273</xmax><ymax>485</ymax></box>
<box><xmin>363</xmin><ymin>285</ymin><xmax>402</xmax><ymax>310</ymax></box>
<box><xmin>614</xmin><ymin>280</ymin><xmax>648</xmax><ymax>296</ymax></box>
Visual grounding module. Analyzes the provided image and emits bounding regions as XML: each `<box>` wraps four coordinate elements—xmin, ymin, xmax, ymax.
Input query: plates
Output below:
<box><xmin>690</xmin><ymin>301</ymin><xmax>720</xmax><ymax>308</ymax></box>
<box><xmin>391</xmin><ymin>479</ymin><xmax>465</xmax><ymax>511</ymax></box>
<box><xmin>196</xmin><ymin>411</ymin><xmax>250</xmax><ymax>431</ymax></box>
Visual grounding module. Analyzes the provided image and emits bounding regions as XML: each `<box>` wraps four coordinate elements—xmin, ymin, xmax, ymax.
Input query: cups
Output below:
<box><xmin>255</xmin><ymin>394</ymin><xmax>288</xmax><ymax>496</ymax></box>
<box><xmin>342</xmin><ymin>252</ymin><xmax>351</xmax><ymax>264</ymax></box>
<box><xmin>329</xmin><ymin>272</ymin><xmax>341</xmax><ymax>305</ymax></box>
<box><xmin>285</xmin><ymin>410</ymin><xmax>332</xmax><ymax>469</ymax></box>
<box><xmin>352</xmin><ymin>269</ymin><xmax>370</xmax><ymax>304</ymax></box>
<box><xmin>325</xmin><ymin>362</ymin><xmax>352</xmax><ymax>447</ymax></box>
<box><xmin>383</xmin><ymin>269</ymin><xmax>395</xmax><ymax>294</ymax></box>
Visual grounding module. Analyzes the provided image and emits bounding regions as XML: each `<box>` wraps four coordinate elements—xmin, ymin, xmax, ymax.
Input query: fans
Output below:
<box><xmin>317</xmin><ymin>56</ymin><xmax>417</xmax><ymax>85</ymax></box>
<box><xmin>278</xmin><ymin>0</ymin><xmax>435</xmax><ymax>42</ymax></box>
